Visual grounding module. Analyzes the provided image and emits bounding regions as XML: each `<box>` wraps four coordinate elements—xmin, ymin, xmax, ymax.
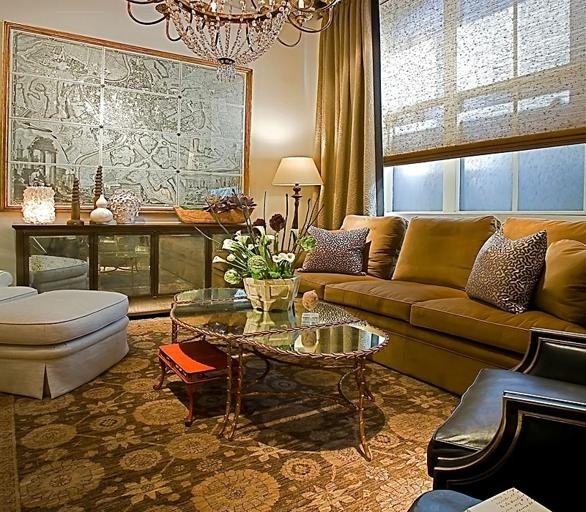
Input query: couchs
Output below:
<box><xmin>29</xmin><ymin>254</ymin><xmax>87</xmax><ymax>293</ymax></box>
<box><xmin>0</xmin><ymin>271</ymin><xmax>130</xmax><ymax>400</ymax></box>
<box><xmin>293</xmin><ymin>215</ymin><xmax>586</xmax><ymax>397</ymax></box>
<box><xmin>425</xmin><ymin>326</ymin><xmax>586</xmax><ymax>488</ymax></box>
<box><xmin>403</xmin><ymin>490</ymin><xmax>481</xmax><ymax>512</ymax></box>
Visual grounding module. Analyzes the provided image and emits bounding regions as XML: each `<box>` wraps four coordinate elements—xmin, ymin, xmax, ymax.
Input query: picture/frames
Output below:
<box><xmin>0</xmin><ymin>20</ymin><xmax>252</xmax><ymax>214</ymax></box>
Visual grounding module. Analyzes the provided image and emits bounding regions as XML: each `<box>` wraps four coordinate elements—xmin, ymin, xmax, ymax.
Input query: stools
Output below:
<box><xmin>155</xmin><ymin>340</ymin><xmax>243</xmax><ymax>426</ymax></box>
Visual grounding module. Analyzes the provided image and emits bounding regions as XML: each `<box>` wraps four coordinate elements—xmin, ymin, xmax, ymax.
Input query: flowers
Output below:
<box><xmin>193</xmin><ymin>187</ymin><xmax>325</xmax><ymax>286</ymax></box>
<box><xmin>204</xmin><ymin>194</ymin><xmax>258</xmax><ymax>214</ymax></box>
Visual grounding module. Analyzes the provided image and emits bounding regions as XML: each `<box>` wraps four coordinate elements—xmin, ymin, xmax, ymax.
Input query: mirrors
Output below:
<box><xmin>93</xmin><ymin>234</ymin><xmax>154</xmax><ymax>299</ymax></box>
<box><xmin>158</xmin><ymin>232</ymin><xmax>208</xmax><ymax>296</ymax></box>
<box><xmin>23</xmin><ymin>232</ymin><xmax>93</xmax><ymax>294</ymax></box>
<box><xmin>208</xmin><ymin>233</ymin><xmax>246</xmax><ymax>291</ymax></box>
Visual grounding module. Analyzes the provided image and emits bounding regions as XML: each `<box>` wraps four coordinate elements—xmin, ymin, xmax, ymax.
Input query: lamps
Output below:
<box><xmin>126</xmin><ymin>0</ymin><xmax>336</xmax><ymax>83</ymax></box>
<box><xmin>21</xmin><ymin>187</ymin><xmax>56</xmax><ymax>225</ymax></box>
<box><xmin>271</xmin><ymin>156</ymin><xmax>324</xmax><ymax>238</ymax></box>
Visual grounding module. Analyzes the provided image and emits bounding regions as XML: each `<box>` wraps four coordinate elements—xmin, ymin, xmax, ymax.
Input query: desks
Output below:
<box><xmin>170</xmin><ymin>287</ymin><xmax>389</xmax><ymax>462</ymax></box>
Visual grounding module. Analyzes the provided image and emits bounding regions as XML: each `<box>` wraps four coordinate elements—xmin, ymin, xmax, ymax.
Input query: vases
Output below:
<box><xmin>109</xmin><ymin>187</ymin><xmax>140</xmax><ymax>225</ymax></box>
<box><xmin>243</xmin><ymin>279</ymin><xmax>298</xmax><ymax>314</ymax></box>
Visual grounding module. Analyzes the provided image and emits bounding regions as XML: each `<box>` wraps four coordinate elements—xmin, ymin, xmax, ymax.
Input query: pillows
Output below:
<box><xmin>535</xmin><ymin>239</ymin><xmax>586</xmax><ymax>322</ymax></box>
<box><xmin>297</xmin><ymin>226</ymin><xmax>369</xmax><ymax>276</ymax></box>
<box><xmin>464</xmin><ymin>226</ymin><xmax>543</xmax><ymax>315</ymax></box>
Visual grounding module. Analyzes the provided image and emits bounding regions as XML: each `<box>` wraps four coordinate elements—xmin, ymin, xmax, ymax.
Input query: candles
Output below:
<box><xmin>298</xmin><ymin>0</ymin><xmax>304</xmax><ymax>27</ymax></box>
